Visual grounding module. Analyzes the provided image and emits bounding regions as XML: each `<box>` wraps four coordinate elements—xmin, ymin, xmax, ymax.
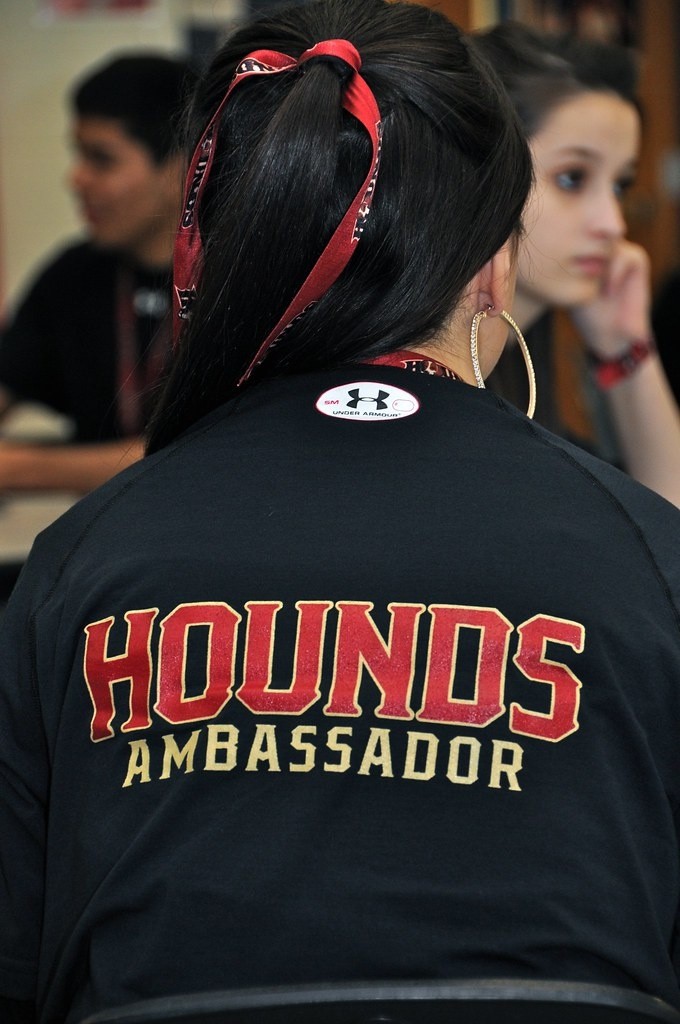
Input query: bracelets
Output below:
<box><xmin>583</xmin><ymin>337</ymin><xmax>658</xmax><ymax>391</ymax></box>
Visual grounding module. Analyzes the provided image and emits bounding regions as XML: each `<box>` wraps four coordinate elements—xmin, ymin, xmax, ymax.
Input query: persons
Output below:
<box><xmin>0</xmin><ymin>0</ymin><xmax>680</xmax><ymax>1024</ymax></box>
<box><xmin>0</xmin><ymin>51</ymin><xmax>207</xmax><ymax>492</ymax></box>
<box><xmin>462</xmin><ymin>20</ymin><xmax>679</xmax><ymax>509</ymax></box>
